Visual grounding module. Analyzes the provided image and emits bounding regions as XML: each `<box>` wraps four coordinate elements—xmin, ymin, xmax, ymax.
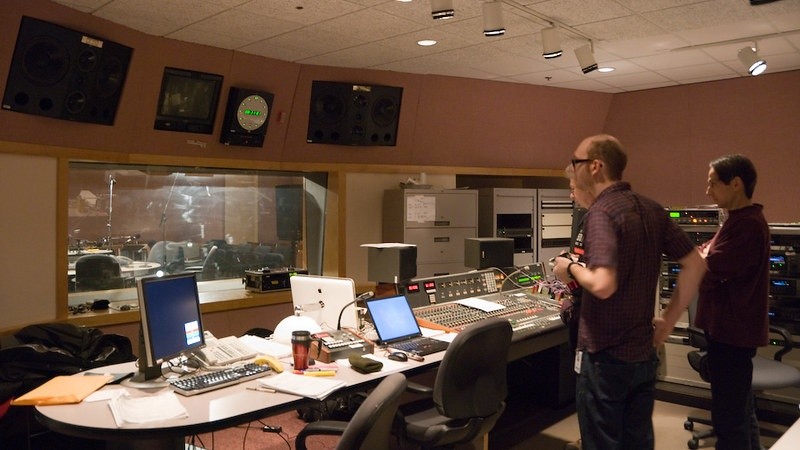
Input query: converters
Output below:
<box><xmin>263</xmin><ymin>425</ymin><xmax>282</xmax><ymax>432</ymax></box>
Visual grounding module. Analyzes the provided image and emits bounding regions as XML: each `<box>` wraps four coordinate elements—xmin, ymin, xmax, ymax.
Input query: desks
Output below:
<box><xmin>67</xmin><ymin>261</ymin><xmax>161</xmax><ymax>288</ymax></box>
<box><xmin>34</xmin><ymin>316</ymin><xmax>564</xmax><ymax>450</ymax></box>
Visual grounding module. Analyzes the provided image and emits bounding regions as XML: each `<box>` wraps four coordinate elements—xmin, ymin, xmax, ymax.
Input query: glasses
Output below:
<box><xmin>571</xmin><ymin>158</ymin><xmax>603</xmax><ymax>170</ymax></box>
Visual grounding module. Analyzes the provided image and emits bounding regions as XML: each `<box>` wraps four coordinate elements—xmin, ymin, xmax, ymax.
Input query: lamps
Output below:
<box><xmin>481</xmin><ymin>0</ymin><xmax>506</xmax><ymax>37</ymax></box>
<box><xmin>573</xmin><ymin>38</ymin><xmax>599</xmax><ymax>75</ymax></box>
<box><xmin>429</xmin><ymin>0</ymin><xmax>454</xmax><ymax>20</ymax></box>
<box><xmin>541</xmin><ymin>22</ymin><xmax>563</xmax><ymax>61</ymax></box>
<box><xmin>738</xmin><ymin>40</ymin><xmax>767</xmax><ymax>79</ymax></box>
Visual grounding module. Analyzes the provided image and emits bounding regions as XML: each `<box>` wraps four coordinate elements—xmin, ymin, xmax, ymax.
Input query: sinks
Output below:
<box><xmin>125</xmin><ymin>265</ymin><xmax>152</xmax><ymax>269</ymax></box>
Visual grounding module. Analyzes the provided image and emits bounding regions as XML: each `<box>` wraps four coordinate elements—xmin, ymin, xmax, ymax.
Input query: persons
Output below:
<box><xmin>688</xmin><ymin>154</ymin><xmax>769</xmax><ymax>450</ymax></box>
<box><xmin>550</xmin><ymin>133</ymin><xmax>708</xmax><ymax>450</ymax></box>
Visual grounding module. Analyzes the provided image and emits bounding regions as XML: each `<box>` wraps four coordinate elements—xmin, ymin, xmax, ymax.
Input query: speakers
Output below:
<box><xmin>307</xmin><ymin>80</ymin><xmax>404</xmax><ymax>146</ymax></box>
<box><xmin>463</xmin><ymin>238</ymin><xmax>514</xmax><ymax>270</ymax></box>
<box><xmin>367</xmin><ymin>244</ymin><xmax>418</xmax><ymax>283</ymax></box>
<box><xmin>1</xmin><ymin>15</ymin><xmax>134</xmax><ymax>126</ymax></box>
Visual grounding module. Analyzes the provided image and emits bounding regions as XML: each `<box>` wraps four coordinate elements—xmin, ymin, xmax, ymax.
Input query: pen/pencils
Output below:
<box><xmin>246</xmin><ymin>386</ymin><xmax>277</xmax><ymax>392</ymax></box>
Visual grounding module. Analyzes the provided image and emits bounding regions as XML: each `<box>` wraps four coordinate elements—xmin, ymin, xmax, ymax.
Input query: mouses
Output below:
<box><xmin>388</xmin><ymin>352</ymin><xmax>407</xmax><ymax>361</ymax></box>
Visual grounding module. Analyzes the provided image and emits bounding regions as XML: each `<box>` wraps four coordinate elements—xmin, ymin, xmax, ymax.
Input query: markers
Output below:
<box><xmin>305</xmin><ymin>371</ymin><xmax>336</xmax><ymax>376</ymax></box>
<box><xmin>388</xmin><ymin>349</ymin><xmax>424</xmax><ymax>361</ymax></box>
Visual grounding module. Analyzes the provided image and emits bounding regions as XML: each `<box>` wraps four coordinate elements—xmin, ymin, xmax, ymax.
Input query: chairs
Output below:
<box><xmin>367</xmin><ymin>316</ymin><xmax>513</xmax><ymax>450</ymax></box>
<box><xmin>146</xmin><ymin>240</ymin><xmax>185</xmax><ymax>272</ymax></box>
<box><xmin>75</xmin><ymin>254</ymin><xmax>121</xmax><ymax>289</ymax></box>
<box><xmin>295</xmin><ymin>372</ymin><xmax>406</xmax><ymax>450</ymax></box>
<box><xmin>683</xmin><ymin>287</ymin><xmax>800</xmax><ymax>450</ymax></box>
<box><xmin>186</xmin><ymin>246</ymin><xmax>218</xmax><ymax>280</ymax></box>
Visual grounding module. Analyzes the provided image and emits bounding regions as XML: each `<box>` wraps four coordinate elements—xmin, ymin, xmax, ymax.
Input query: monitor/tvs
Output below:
<box><xmin>119</xmin><ymin>272</ymin><xmax>206</xmax><ymax>388</ymax></box>
<box><xmin>154</xmin><ymin>67</ymin><xmax>224</xmax><ymax>134</ymax></box>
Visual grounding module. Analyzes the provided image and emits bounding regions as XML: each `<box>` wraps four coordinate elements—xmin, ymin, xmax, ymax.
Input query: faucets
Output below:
<box><xmin>138</xmin><ymin>247</ymin><xmax>148</xmax><ymax>265</ymax></box>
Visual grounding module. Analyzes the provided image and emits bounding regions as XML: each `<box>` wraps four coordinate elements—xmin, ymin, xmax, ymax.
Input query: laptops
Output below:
<box><xmin>365</xmin><ymin>294</ymin><xmax>450</xmax><ymax>356</ymax></box>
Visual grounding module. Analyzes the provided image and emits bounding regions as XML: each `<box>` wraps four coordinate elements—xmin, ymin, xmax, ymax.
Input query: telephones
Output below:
<box><xmin>191</xmin><ymin>330</ymin><xmax>256</xmax><ymax>367</ymax></box>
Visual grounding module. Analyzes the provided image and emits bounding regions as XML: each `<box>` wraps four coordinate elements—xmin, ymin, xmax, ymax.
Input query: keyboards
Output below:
<box><xmin>170</xmin><ymin>361</ymin><xmax>272</xmax><ymax>396</ymax></box>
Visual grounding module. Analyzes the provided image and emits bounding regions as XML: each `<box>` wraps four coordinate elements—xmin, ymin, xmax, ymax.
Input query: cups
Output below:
<box><xmin>291</xmin><ymin>331</ymin><xmax>322</xmax><ymax>370</ymax></box>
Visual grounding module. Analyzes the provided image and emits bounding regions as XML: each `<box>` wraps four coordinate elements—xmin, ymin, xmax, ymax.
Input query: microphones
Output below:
<box><xmin>499</xmin><ymin>266</ymin><xmax>531</xmax><ymax>301</ymax></box>
<box><xmin>337</xmin><ymin>291</ymin><xmax>374</xmax><ymax>330</ymax></box>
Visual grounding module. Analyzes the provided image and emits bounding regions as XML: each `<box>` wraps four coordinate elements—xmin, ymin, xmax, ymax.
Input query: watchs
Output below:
<box><xmin>567</xmin><ymin>262</ymin><xmax>584</xmax><ymax>279</ymax></box>
